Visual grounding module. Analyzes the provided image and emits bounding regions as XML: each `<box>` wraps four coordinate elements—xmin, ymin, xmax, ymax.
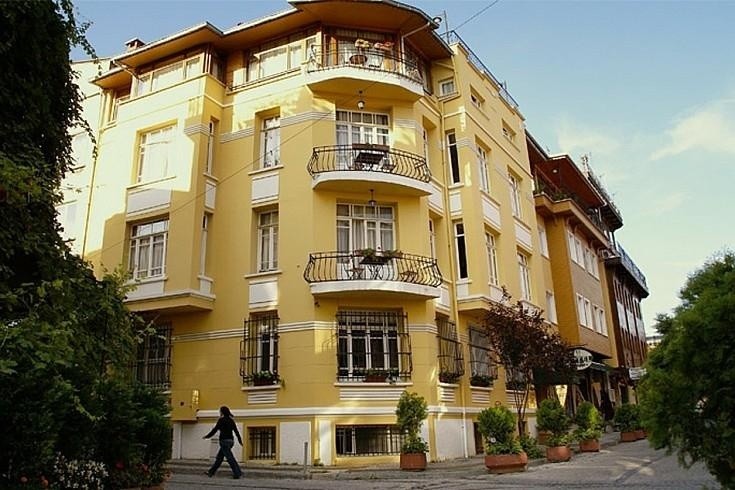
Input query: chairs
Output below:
<box><xmin>341</xmin><ymin>256</ymin><xmax>364</xmax><ymax>280</ymax></box>
<box><xmin>398</xmin><ymin>264</ymin><xmax>419</xmax><ymax>281</ymax></box>
<box><xmin>381</xmin><ymin>155</ymin><xmax>397</xmax><ymax>173</ymax></box>
<box><xmin>343</xmin><ymin>152</ymin><xmax>364</xmax><ymax>171</ymax></box>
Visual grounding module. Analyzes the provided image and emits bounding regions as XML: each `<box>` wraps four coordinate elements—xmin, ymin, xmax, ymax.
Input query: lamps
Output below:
<box><xmin>369</xmin><ymin>188</ymin><xmax>376</xmax><ymax>206</ymax></box>
<box><xmin>357</xmin><ymin>90</ymin><xmax>364</xmax><ymax>110</ymax></box>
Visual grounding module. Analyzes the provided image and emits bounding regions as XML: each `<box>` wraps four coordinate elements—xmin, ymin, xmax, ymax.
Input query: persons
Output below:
<box><xmin>202</xmin><ymin>406</ymin><xmax>245</xmax><ymax>479</ymax></box>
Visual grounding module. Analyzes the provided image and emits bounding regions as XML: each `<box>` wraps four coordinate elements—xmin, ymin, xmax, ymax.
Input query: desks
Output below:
<box><xmin>358</xmin><ymin>260</ymin><xmax>386</xmax><ymax>280</ymax></box>
<box><xmin>354</xmin><ymin>152</ymin><xmax>384</xmax><ymax>170</ymax></box>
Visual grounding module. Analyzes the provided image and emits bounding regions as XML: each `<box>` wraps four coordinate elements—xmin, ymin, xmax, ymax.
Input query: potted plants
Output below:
<box><xmin>365</xmin><ymin>368</ymin><xmax>389</xmax><ymax>382</ymax></box>
<box><xmin>253</xmin><ymin>370</ymin><xmax>276</xmax><ymax>386</ymax></box>
<box><xmin>395</xmin><ymin>390</ymin><xmax>430</xmax><ymax>470</ymax></box>
<box><xmin>476</xmin><ymin>398</ymin><xmax>644</xmax><ymax>473</ymax></box>
